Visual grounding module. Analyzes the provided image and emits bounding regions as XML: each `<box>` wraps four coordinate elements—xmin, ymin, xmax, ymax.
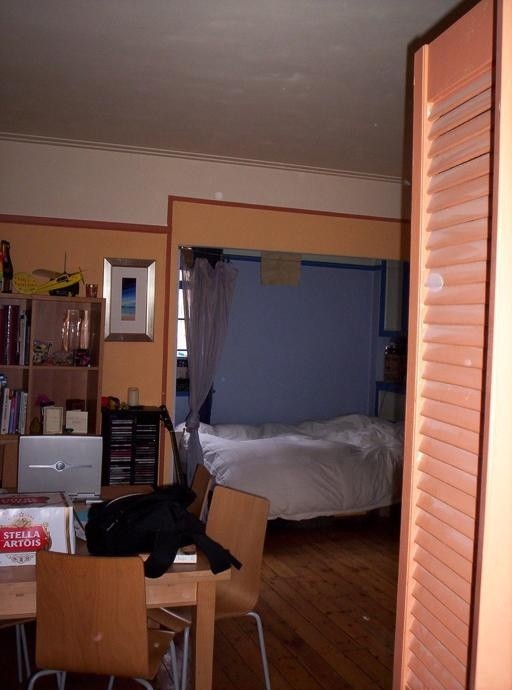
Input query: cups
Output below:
<box><xmin>128</xmin><ymin>387</ymin><xmax>139</xmax><ymax>408</ymax></box>
<box><xmin>87</xmin><ymin>283</ymin><xmax>96</xmax><ymax>297</ymax></box>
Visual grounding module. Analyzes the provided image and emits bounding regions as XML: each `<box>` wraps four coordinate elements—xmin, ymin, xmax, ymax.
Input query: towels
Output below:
<box><xmin>260</xmin><ymin>252</ymin><xmax>303</xmax><ymax>288</ymax></box>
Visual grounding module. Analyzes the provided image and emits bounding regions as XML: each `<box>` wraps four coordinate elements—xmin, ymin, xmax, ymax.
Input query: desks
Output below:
<box><xmin>2</xmin><ymin>493</ymin><xmax>243</xmax><ymax>690</ymax></box>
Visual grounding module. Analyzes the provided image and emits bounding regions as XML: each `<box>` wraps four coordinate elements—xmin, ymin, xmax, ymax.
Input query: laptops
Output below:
<box><xmin>15</xmin><ymin>434</ymin><xmax>104</xmax><ymax>503</ymax></box>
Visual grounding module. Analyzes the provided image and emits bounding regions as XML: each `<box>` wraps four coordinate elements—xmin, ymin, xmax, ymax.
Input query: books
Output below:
<box><xmin>0</xmin><ymin>387</ymin><xmax>28</xmax><ymax>435</ymax></box>
<box><xmin>0</xmin><ymin>305</ymin><xmax>31</xmax><ymax>366</ymax></box>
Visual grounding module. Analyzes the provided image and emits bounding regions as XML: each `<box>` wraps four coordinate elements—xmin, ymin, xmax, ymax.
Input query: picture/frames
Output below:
<box><xmin>102</xmin><ymin>256</ymin><xmax>156</xmax><ymax>342</ymax></box>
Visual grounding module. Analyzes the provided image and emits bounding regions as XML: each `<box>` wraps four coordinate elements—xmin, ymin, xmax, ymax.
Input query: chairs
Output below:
<box><xmin>147</xmin><ymin>483</ymin><xmax>274</xmax><ymax>689</ymax></box>
<box><xmin>23</xmin><ymin>549</ymin><xmax>177</xmax><ymax>688</ymax></box>
<box><xmin>180</xmin><ymin>465</ymin><xmax>212</xmax><ymax>524</ymax></box>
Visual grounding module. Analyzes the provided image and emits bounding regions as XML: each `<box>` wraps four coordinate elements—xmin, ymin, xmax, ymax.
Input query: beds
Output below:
<box><xmin>160</xmin><ymin>380</ymin><xmax>407</xmax><ymax>527</ymax></box>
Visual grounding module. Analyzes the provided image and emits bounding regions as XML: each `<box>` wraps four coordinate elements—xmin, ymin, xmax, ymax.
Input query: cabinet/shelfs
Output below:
<box><xmin>102</xmin><ymin>405</ymin><xmax>161</xmax><ymax>488</ymax></box>
<box><xmin>1</xmin><ymin>291</ymin><xmax>107</xmax><ymax>494</ymax></box>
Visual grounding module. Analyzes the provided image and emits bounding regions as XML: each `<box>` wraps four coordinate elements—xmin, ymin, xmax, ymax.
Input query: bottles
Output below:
<box><xmin>80</xmin><ymin>310</ymin><xmax>90</xmax><ymax>349</ymax></box>
<box><xmin>0</xmin><ymin>239</ymin><xmax>13</xmax><ymax>293</ymax></box>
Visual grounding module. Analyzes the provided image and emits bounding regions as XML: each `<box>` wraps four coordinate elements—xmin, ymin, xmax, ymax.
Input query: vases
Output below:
<box><xmin>127</xmin><ymin>387</ymin><xmax>139</xmax><ymax>406</ymax></box>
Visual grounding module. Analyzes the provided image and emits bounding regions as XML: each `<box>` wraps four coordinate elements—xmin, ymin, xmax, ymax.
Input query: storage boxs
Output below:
<box><xmin>3</xmin><ymin>495</ymin><xmax>76</xmax><ymax>567</ymax></box>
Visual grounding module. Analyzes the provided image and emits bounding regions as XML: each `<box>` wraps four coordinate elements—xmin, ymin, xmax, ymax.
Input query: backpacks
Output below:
<box><xmin>86</xmin><ymin>484</ymin><xmax>242</xmax><ymax>579</ymax></box>
